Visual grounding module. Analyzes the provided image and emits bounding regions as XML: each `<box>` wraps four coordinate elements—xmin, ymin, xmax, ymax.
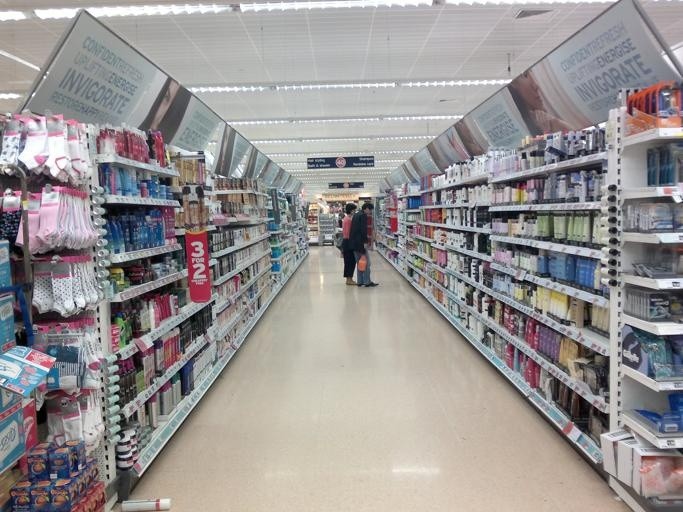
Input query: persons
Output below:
<box><xmin>348</xmin><ymin>202</ymin><xmax>379</xmax><ymax>289</ymax></box>
<box><xmin>341</xmin><ymin>203</ymin><xmax>358</xmax><ymax>286</ymax></box>
<box><xmin>338</xmin><ymin>209</ymin><xmax>345</xmax><ymax>228</ymax></box>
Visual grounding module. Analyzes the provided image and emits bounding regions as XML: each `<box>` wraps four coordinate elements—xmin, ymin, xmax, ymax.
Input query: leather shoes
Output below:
<box><xmin>365</xmin><ymin>282</ymin><xmax>379</xmax><ymax>287</ymax></box>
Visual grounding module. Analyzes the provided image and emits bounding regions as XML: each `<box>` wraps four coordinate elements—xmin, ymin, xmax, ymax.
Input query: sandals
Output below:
<box><xmin>346</xmin><ymin>280</ymin><xmax>356</xmax><ymax>284</ymax></box>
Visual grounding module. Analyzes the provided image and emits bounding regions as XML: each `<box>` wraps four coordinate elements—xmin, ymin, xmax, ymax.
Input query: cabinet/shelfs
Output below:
<box><xmin>374</xmin><ymin>88</ymin><xmax>683</xmax><ymax>512</ymax></box>
<box><xmin>84</xmin><ymin>124</ymin><xmax>310</xmax><ymax>502</ymax></box>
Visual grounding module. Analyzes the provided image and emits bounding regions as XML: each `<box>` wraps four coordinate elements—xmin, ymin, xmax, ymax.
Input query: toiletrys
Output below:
<box><xmin>94</xmin><ymin>122</ymin><xmax>276</xmax><ymax>475</ymax></box>
<box><xmin>410</xmin><ymin>131</ymin><xmax>616</xmax><ymax>448</ymax></box>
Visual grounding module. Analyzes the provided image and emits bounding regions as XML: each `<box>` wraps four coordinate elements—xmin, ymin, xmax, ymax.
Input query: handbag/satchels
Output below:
<box><xmin>336</xmin><ymin>236</ymin><xmax>343</xmax><ymax>252</ymax></box>
